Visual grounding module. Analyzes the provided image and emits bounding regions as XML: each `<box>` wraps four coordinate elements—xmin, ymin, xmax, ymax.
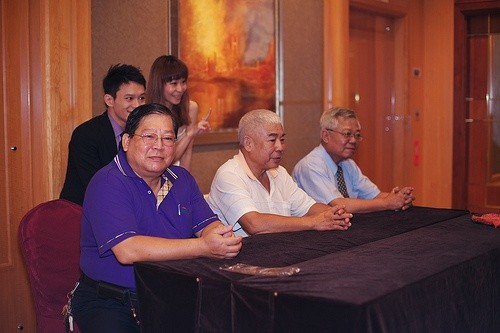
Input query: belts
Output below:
<box><xmin>82</xmin><ymin>275</ymin><xmax>95</xmax><ymax>288</ymax></box>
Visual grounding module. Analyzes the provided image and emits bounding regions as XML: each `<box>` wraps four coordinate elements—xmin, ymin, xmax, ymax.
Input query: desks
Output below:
<box><xmin>134</xmin><ymin>206</ymin><xmax>499</xmax><ymax>333</ymax></box>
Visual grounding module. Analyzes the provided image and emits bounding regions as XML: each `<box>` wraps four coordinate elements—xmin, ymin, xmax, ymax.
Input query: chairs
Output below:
<box><xmin>19</xmin><ymin>199</ymin><xmax>89</xmax><ymax>333</ymax></box>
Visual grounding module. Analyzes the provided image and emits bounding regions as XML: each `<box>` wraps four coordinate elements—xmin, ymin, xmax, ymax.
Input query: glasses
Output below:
<box><xmin>326</xmin><ymin>128</ymin><xmax>363</xmax><ymax>140</ymax></box>
<box><xmin>133</xmin><ymin>132</ymin><xmax>176</xmax><ymax>147</ymax></box>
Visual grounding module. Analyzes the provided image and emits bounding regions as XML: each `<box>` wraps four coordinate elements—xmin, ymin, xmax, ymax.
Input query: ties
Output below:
<box><xmin>118</xmin><ymin>132</ymin><xmax>127</xmax><ymax>149</ymax></box>
<box><xmin>336</xmin><ymin>167</ymin><xmax>349</xmax><ymax>198</ymax></box>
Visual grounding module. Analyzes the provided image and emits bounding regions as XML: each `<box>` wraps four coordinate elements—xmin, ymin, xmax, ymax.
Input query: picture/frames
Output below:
<box><xmin>167</xmin><ymin>0</ymin><xmax>285</xmax><ymax>147</ymax></box>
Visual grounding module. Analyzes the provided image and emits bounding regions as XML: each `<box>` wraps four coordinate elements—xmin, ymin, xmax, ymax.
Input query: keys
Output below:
<box><xmin>60</xmin><ymin>297</ymin><xmax>74</xmax><ymax>333</ymax></box>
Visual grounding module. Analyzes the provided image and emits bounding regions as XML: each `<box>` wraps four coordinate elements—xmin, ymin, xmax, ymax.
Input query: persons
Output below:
<box><xmin>290</xmin><ymin>106</ymin><xmax>416</xmax><ymax>213</ymax></box>
<box><xmin>59</xmin><ymin>63</ymin><xmax>147</xmax><ymax>208</ymax></box>
<box><xmin>145</xmin><ymin>55</ymin><xmax>199</xmax><ymax>173</ymax></box>
<box><xmin>206</xmin><ymin>108</ymin><xmax>353</xmax><ymax>239</ymax></box>
<box><xmin>71</xmin><ymin>102</ymin><xmax>242</xmax><ymax>333</ymax></box>
